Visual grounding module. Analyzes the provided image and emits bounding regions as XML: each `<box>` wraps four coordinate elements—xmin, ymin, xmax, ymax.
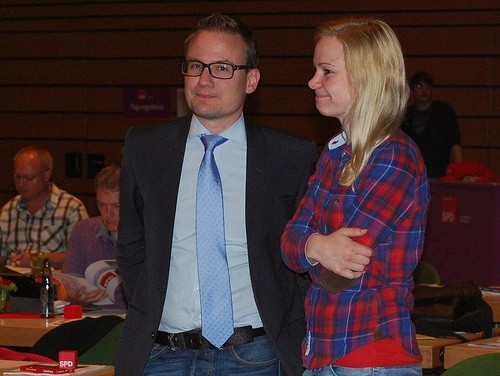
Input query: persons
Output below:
<box><xmin>400</xmin><ymin>70</ymin><xmax>464</xmax><ymax>179</ymax></box>
<box><xmin>0</xmin><ymin>145</ymin><xmax>90</xmax><ymax>275</ymax></box>
<box><xmin>280</xmin><ymin>16</ymin><xmax>432</xmax><ymax>376</ymax></box>
<box><xmin>62</xmin><ymin>166</ymin><xmax>128</xmax><ymax>311</ymax></box>
<box><xmin>114</xmin><ymin>11</ymin><xmax>320</xmax><ymax>376</ymax></box>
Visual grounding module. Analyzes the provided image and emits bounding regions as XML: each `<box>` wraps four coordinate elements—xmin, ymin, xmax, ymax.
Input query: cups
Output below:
<box><xmin>31</xmin><ymin>253</ymin><xmax>48</xmax><ymax>276</ymax></box>
<box><xmin>0</xmin><ymin>284</ymin><xmax>7</xmax><ymax>313</ymax></box>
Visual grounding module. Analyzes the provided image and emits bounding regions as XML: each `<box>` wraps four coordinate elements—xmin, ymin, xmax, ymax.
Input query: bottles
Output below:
<box><xmin>40</xmin><ymin>258</ymin><xmax>54</xmax><ymax>318</ymax></box>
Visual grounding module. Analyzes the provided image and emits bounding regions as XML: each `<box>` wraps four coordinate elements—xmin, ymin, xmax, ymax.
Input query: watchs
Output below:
<box><xmin>39</xmin><ymin>252</ymin><xmax>49</xmax><ymax>267</ymax></box>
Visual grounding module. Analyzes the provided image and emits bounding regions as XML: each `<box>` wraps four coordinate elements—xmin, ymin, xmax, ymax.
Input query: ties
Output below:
<box><xmin>196</xmin><ymin>135</ymin><xmax>234</xmax><ymax>348</ymax></box>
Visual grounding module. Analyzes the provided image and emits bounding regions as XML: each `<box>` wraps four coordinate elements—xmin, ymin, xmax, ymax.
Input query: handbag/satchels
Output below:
<box><xmin>412</xmin><ymin>282</ymin><xmax>494</xmax><ymax>342</ymax></box>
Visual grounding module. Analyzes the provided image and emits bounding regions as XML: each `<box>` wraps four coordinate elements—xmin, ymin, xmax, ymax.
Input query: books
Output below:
<box><xmin>52</xmin><ymin>260</ymin><xmax>122</xmax><ymax>306</ymax></box>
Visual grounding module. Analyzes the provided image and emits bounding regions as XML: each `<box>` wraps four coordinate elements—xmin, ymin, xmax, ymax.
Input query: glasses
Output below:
<box><xmin>413</xmin><ymin>83</ymin><xmax>429</xmax><ymax>90</ymax></box>
<box><xmin>180</xmin><ymin>60</ymin><xmax>252</xmax><ymax>79</ymax></box>
<box><xmin>13</xmin><ymin>170</ymin><xmax>49</xmax><ymax>182</ymax></box>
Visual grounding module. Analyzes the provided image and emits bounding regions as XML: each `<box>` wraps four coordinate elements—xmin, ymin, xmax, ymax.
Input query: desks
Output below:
<box><xmin>415</xmin><ymin>286</ymin><xmax>500</xmax><ymax>376</ymax></box>
<box><xmin>0</xmin><ymin>288</ymin><xmax>127</xmax><ymax>376</ymax></box>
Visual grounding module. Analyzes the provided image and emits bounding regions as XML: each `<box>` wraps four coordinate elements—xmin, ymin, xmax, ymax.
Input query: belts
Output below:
<box><xmin>157</xmin><ymin>325</ymin><xmax>266</xmax><ymax>349</ymax></box>
